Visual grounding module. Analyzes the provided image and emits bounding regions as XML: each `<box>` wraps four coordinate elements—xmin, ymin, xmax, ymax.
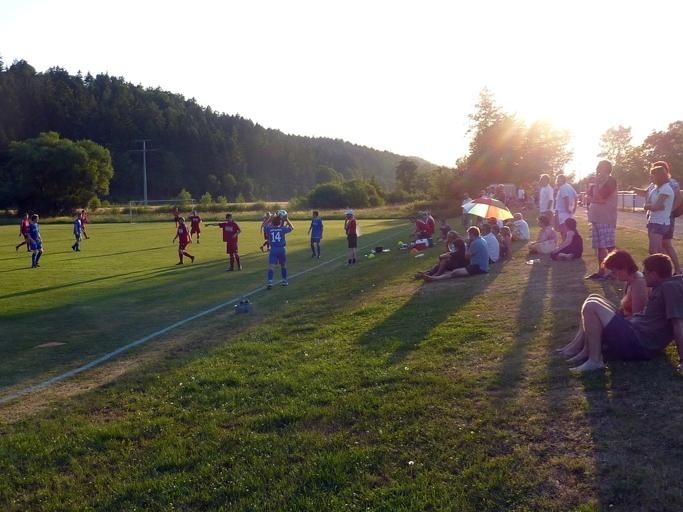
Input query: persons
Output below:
<box><xmin>306</xmin><ymin>210</ymin><xmax>324</xmax><ymax>259</ymax></box>
<box><xmin>460</xmin><ymin>183</ymin><xmax>506</xmax><ymax>226</ymax></box>
<box><xmin>409</xmin><ymin>210</ymin><xmax>435</xmax><ymax>242</ymax></box>
<box><xmin>344</xmin><ymin>212</ymin><xmax>358</xmax><ymax>264</ymax></box>
<box><xmin>432</xmin><ymin>217</ymin><xmax>452</xmax><ymax>245</ymax></box>
<box><xmin>26</xmin><ymin>212</ymin><xmax>43</xmax><ymax>268</ymax></box>
<box><xmin>15</xmin><ymin>212</ymin><xmax>30</xmax><ymax>253</ymax></box>
<box><xmin>583</xmin><ymin>161</ymin><xmax>617</xmax><ymax>280</ymax></box>
<box><xmin>506</xmin><ymin>185</ymin><xmax>537</xmax><ymax>214</ymax></box>
<box><xmin>478</xmin><ymin>213</ymin><xmax>529</xmax><ymax>263</ymax></box>
<box><xmin>622</xmin><ymin>160</ymin><xmax>682</xmax><ymax>276</ymax></box>
<box><xmin>346</xmin><ymin>205</ymin><xmax>353</xmax><ymax>214</ymax></box>
<box><xmin>71</xmin><ymin>214</ymin><xmax>81</xmax><ymax>252</ymax></box>
<box><xmin>77</xmin><ymin>210</ymin><xmax>90</xmax><ymax>238</ymax></box>
<box><xmin>173</xmin><ymin>207</ymin><xmax>295</xmax><ymax>289</ymax></box>
<box><xmin>528</xmin><ymin>173</ymin><xmax>584</xmax><ymax>261</ymax></box>
<box><xmin>413</xmin><ymin>227</ymin><xmax>490</xmax><ymax>282</ymax></box>
<box><xmin>552</xmin><ymin>249</ymin><xmax>682</xmax><ymax>378</ymax></box>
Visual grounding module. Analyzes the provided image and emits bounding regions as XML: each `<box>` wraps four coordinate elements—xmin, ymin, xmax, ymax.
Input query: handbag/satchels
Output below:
<box><xmin>673</xmin><ymin>205</ymin><xmax>683</xmax><ymax>217</ymax></box>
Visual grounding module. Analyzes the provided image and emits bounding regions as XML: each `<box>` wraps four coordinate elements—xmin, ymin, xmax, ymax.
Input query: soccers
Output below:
<box><xmin>277</xmin><ymin>210</ymin><xmax>287</xmax><ymax>219</ymax></box>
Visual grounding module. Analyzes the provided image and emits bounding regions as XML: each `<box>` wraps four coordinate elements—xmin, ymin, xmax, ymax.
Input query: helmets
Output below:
<box><xmin>345</xmin><ymin>210</ymin><xmax>353</xmax><ymax>214</ymax></box>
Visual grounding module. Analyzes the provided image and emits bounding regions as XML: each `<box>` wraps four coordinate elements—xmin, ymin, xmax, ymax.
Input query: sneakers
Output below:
<box><xmin>226</xmin><ymin>268</ymin><xmax>232</xmax><ymax>271</ymax></box>
<box><xmin>72</xmin><ymin>246</ymin><xmax>75</xmax><ymax>250</ymax></box>
<box><xmin>191</xmin><ymin>256</ymin><xmax>194</xmax><ymax>262</ymax></box>
<box><xmin>584</xmin><ymin>273</ymin><xmax>603</xmax><ymax>279</ymax></box>
<box><xmin>602</xmin><ymin>273</ymin><xmax>617</xmax><ymax>281</ymax></box>
<box><xmin>76</xmin><ymin>249</ymin><xmax>80</xmax><ymax>251</ymax></box>
<box><xmin>238</xmin><ymin>266</ymin><xmax>242</xmax><ymax>270</ymax></box>
<box><xmin>176</xmin><ymin>262</ymin><xmax>183</xmax><ymax>265</ymax></box>
